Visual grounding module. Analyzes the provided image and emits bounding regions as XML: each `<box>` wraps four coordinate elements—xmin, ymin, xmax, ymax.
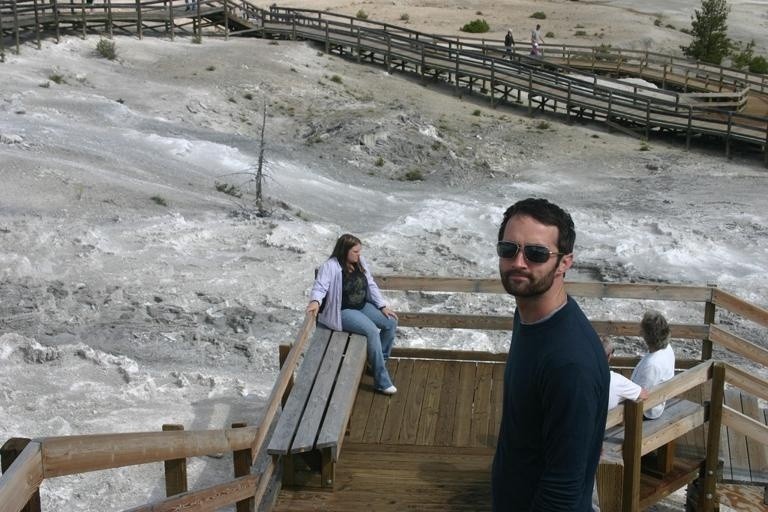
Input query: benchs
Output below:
<box><xmin>598</xmin><ymin>398</ymin><xmax>710</xmax><ymax>473</ymax></box>
<box><xmin>267</xmin><ymin>323</ymin><xmax>367</xmax><ymax>489</ymax></box>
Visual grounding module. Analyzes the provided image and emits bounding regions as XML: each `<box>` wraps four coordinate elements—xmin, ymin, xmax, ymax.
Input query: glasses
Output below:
<box><xmin>497</xmin><ymin>241</ymin><xmax>564</xmax><ymax>263</ymax></box>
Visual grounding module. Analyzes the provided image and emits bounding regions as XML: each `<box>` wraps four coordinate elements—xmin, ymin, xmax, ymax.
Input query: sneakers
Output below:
<box><xmin>379</xmin><ymin>385</ymin><xmax>396</xmax><ymax>394</ymax></box>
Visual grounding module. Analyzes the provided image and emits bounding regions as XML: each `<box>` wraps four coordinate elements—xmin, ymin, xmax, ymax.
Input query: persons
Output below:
<box><xmin>528</xmin><ymin>24</ymin><xmax>545</xmax><ymax>56</ymax></box>
<box><xmin>627</xmin><ymin>309</ymin><xmax>676</xmax><ymax>421</ymax></box>
<box><xmin>490</xmin><ymin>197</ymin><xmax>612</xmax><ymax>511</ymax></box>
<box><xmin>595</xmin><ymin>334</ymin><xmax>648</xmax><ymax>411</ymax></box>
<box><xmin>306</xmin><ymin>233</ymin><xmax>399</xmax><ymax>395</ymax></box>
<box><xmin>184</xmin><ymin>0</ymin><xmax>197</xmax><ymax>13</ymax></box>
<box><xmin>502</xmin><ymin>29</ymin><xmax>516</xmax><ymax>61</ymax></box>
